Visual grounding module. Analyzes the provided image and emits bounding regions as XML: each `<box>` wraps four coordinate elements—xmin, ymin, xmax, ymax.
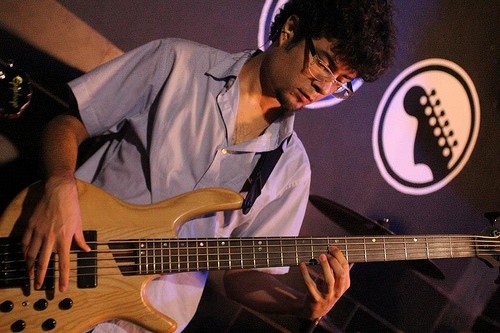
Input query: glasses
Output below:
<box><xmin>305</xmin><ymin>36</ymin><xmax>354</xmax><ymax>101</ymax></box>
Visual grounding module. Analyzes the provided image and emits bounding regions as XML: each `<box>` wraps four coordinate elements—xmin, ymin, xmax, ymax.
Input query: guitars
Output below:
<box><xmin>0</xmin><ymin>176</ymin><xmax>500</xmax><ymax>333</ymax></box>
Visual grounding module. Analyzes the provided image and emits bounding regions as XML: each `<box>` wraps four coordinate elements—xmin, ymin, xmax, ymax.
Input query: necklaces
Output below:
<box><xmin>233</xmin><ymin>110</ymin><xmax>270</xmax><ymax>145</ymax></box>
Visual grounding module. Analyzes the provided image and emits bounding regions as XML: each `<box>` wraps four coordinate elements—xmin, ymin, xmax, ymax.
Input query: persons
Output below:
<box><xmin>21</xmin><ymin>0</ymin><xmax>397</xmax><ymax>333</ymax></box>
